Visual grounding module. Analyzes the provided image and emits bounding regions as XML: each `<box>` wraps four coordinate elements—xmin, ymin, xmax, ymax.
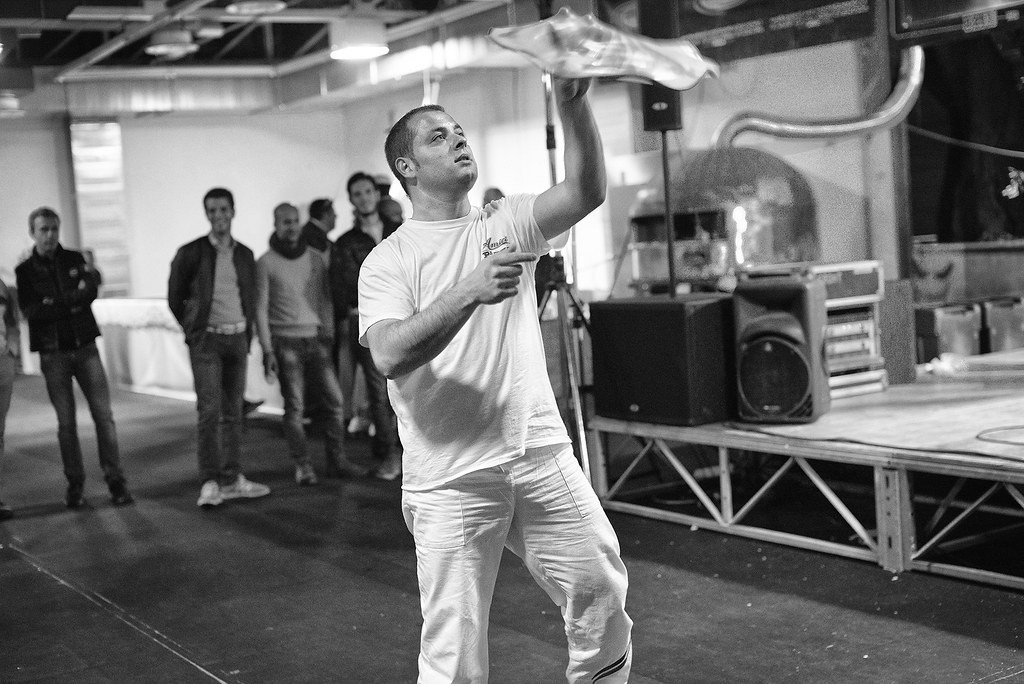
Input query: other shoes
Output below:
<box><xmin>328</xmin><ymin>458</ymin><xmax>370</xmax><ymax>479</ymax></box>
<box><xmin>294</xmin><ymin>461</ymin><xmax>318</xmax><ymax>487</ymax></box>
<box><xmin>110</xmin><ymin>482</ymin><xmax>132</xmax><ymax>506</ymax></box>
<box><xmin>62</xmin><ymin>484</ymin><xmax>85</xmax><ymax>507</ymax></box>
<box><xmin>372</xmin><ymin>452</ymin><xmax>403</xmax><ymax>480</ymax></box>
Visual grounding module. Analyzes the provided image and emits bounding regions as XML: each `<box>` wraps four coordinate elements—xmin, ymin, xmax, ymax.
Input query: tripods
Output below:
<box><xmin>536</xmin><ymin>0</ymin><xmax>664</xmax><ymax>486</ymax></box>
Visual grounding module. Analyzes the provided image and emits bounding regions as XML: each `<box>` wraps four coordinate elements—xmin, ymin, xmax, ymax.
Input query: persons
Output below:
<box><xmin>358</xmin><ymin>8</ymin><xmax>633</xmax><ymax>683</ymax></box>
<box><xmin>0</xmin><ymin>206</ymin><xmax>133</xmax><ymax>508</ymax></box>
<box><xmin>167</xmin><ymin>172</ymin><xmax>404</xmax><ymax>507</ymax></box>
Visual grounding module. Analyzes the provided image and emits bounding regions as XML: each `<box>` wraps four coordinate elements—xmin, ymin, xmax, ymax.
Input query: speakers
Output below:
<box><xmin>733</xmin><ymin>268</ymin><xmax>832</xmax><ymax>425</ymax></box>
<box><xmin>589</xmin><ymin>290</ymin><xmax>733</xmax><ymax>425</ymax></box>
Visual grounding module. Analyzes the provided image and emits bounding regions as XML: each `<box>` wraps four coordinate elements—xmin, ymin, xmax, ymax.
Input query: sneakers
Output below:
<box><xmin>220</xmin><ymin>472</ymin><xmax>271</xmax><ymax>500</ymax></box>
<box><xmin>197</xmin><ymin>480</ymin><xmax>224</xmax><ymax>508</ymax></box>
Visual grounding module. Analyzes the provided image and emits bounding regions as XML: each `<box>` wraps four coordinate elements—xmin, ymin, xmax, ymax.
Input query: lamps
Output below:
<box><xmin>327</xmin><ymin>15</ymin><xmax>389</xmax><ymax>61</ymax></box>
